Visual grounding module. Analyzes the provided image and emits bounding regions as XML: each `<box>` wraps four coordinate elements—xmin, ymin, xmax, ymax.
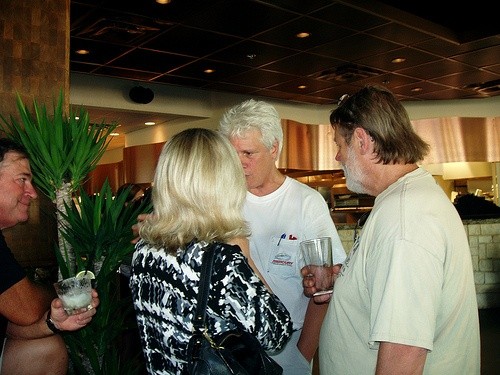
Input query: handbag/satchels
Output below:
<box><xmin>184</xmin><ymin>243</ymin><xmax>284</xmax><ymax>375</ymax></box>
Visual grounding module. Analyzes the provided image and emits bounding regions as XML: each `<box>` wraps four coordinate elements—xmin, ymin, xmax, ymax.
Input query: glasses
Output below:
<box><xmin>338</xmin><ymin>93</ymin><xmax>355</xmax><ymax>118</ymax></box>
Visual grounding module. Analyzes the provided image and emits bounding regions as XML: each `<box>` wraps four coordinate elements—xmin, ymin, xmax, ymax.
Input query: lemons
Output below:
<box><xmin>76</xmin><ymin>271</ymin><xmax>95</xmax><ymax>279</ymax></box>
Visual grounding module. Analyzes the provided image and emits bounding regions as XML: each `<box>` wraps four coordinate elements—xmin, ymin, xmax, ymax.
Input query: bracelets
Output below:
<box><xmin>46</xmin><ymin>308</ymin><xmax>63</xmax><ymax>336</ymax></box>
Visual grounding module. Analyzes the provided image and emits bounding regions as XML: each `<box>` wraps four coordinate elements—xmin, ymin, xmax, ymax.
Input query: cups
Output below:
<box><xmin>300</xmin><ymin>237</ymin><xmax>334</xmax><ymax>297</ymax></box>
<box><xmin>53</xmin><ymin>275</ymin><xmax>94</xmax><ymax>316</ymax></box>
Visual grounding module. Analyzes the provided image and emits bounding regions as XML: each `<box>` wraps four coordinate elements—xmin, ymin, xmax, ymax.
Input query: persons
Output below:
<box><xmin>298</xmin><ymin>83</ymin><xmax>483</xmax><ymax>375</ymax></box>
<box><xmin>131</xmin><ymin>99</ymin><xmax>349</xmax><ymax>375</ymax></box>
<box><xmin>0</xmin><ymin>135</ymin><xmax>103</xmax><ymax>375</ymax></box>
<box><xmin>128</xmin><ymin>126</ymin><xmax>294</xmax><ymax>375</ymax></box>
